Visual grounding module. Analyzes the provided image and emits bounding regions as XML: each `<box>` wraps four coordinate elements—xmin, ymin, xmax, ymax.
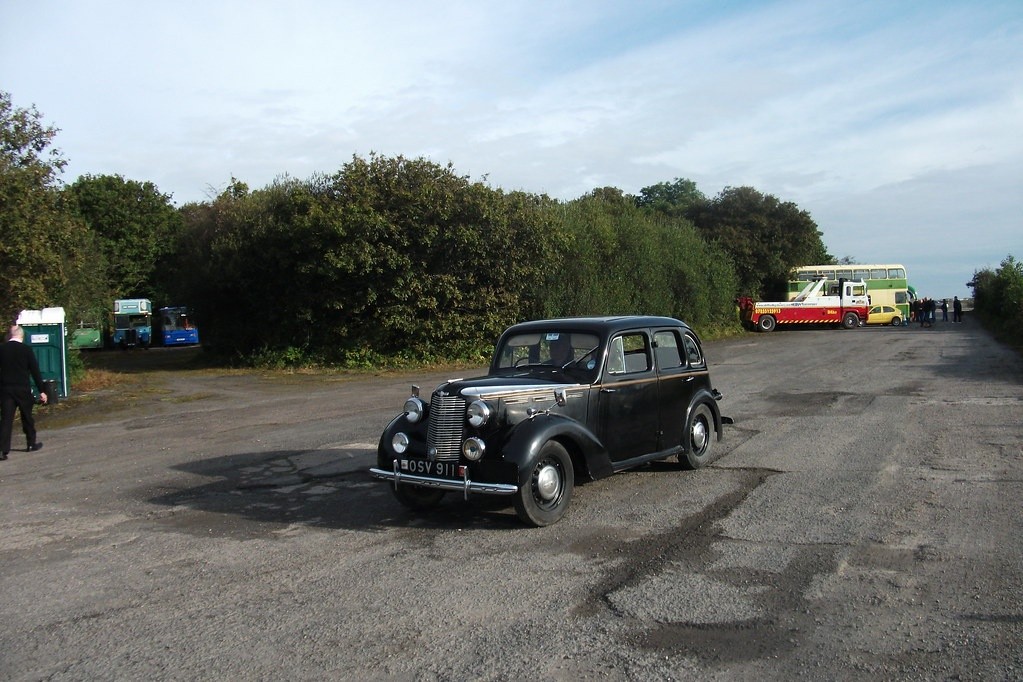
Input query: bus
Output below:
<box><xmin>781</xmin><ymin>263</ymin><xmax>910</xmax><ymax>320</ymax></box>
<box><xmin>111</xmin><ymin>298</ymin><xmax>152</xmax><ymax>346</ymax></box>
<box><xmin>159</xmin><ymin>306</ymin><xmax>201</xmax><ymax>345</ymax></box>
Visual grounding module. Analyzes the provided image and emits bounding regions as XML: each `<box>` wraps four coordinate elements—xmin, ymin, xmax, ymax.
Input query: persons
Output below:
<box><xmin>942</xmin><ymin>299</ymin><xmax>948</xmax><ymax>321</ymax></box>
<box><xmin>909</xmin><ymin>297</ymin><xmax>937</xmax><ymax>328</ymax></box>
<box><xmin>952</xmin><ymin>296</ymin><xmax>962</xmax><ymax>323</ymax></box>
<box><xmin>544</xmin><ymin>335</ymin><xmax>581</xmax><ymax>370</ymax></box>
<box><xmin>0</xmin><ymin>325</ymin><xmax>48</xmax><ymax>459</ymax></box>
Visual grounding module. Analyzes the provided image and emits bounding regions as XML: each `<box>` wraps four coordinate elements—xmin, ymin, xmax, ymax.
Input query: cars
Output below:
<box><xmin>866</xmin><ymin>305</ymin><xmax>905</xmax><ymax>326</ymax></box>
<box><xmin>368</xmin><ymin>314</ymin><xmax>736</xmax><ymax>527</ymax></box>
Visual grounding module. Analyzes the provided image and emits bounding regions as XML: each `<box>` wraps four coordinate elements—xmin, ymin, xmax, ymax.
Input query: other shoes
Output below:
<box><xmin>27</xmin><ymin>442</ymin><xmax>43</xmax><ymax>452</ymax></box>
<box><xmin>0</xmin><ymin>451</ymin><xmax>7</xmax><ymax>460</ymax></box>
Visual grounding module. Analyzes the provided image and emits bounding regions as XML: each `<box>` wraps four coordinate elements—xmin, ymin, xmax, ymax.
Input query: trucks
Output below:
<box><xmin>742</xmin><ymin>277</ymin><xmax>871</xmax><ymax>328</ymax></box>
